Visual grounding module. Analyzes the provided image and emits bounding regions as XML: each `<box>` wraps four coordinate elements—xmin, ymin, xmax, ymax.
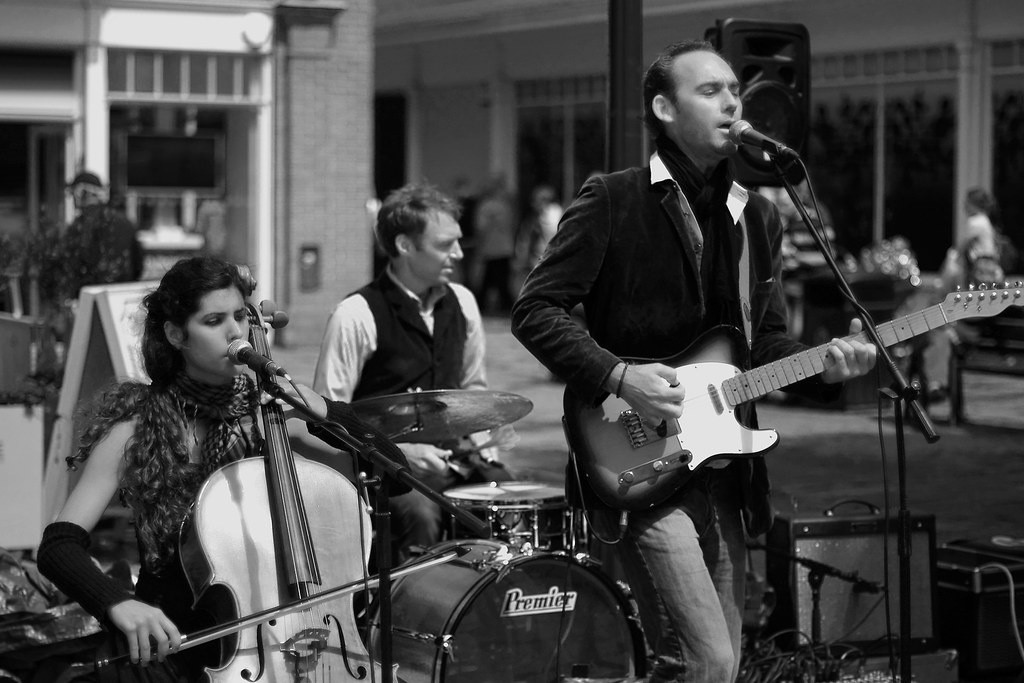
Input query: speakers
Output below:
<box><xmin>766</xmin><ymin>507</ymin><xmax>942</xmax><ymax>661</ymax></box>
<box><xmin>703</xmin><ymin>17</ymin><xmax>812</xmax><ymax>189</ymax></box>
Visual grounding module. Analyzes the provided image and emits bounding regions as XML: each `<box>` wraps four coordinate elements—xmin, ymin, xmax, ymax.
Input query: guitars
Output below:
<box><xmin>576</xmin><ymin>274</ymin><xmax>1024</xmax><ymax>521</ymax></box>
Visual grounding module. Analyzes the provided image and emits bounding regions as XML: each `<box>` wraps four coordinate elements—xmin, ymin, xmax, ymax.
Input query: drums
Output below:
<box><xmin>373</xmin><ymin>466</ymin><xmax>656</xmax><ymax>683</ymax></box>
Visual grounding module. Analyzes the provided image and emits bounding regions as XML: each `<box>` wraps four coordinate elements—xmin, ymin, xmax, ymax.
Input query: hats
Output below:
<box><xmin>63</xmin><ymin>172</ymin><xmax>102</xmax><ymax>188</ymax></box>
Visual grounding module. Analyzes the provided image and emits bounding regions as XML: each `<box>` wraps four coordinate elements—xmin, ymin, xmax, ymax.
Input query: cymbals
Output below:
<box><xmin>347</xmin><ymin>389</ymin><xmax>533</xmax><ymax>442</ymax></box>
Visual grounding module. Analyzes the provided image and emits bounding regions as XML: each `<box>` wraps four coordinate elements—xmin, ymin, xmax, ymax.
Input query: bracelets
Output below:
<box><xmin>616</xmin><ymin>361</ymin><xmax>630</xmax><ymax>400</ymax></box>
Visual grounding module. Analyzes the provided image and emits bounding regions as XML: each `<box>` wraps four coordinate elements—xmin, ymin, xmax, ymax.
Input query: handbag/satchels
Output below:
<box><xmin>972</xmin><ymin>257</ymin><xmax>1006</xmax><ymax>288</ymax></box>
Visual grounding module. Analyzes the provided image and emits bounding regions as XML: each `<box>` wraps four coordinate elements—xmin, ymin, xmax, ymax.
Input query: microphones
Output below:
<box><xmin>729</xmin><ymin>119</ymin><xmax>801</xmax><ymax>159</ymax></box>
<box><xmin>227</xmin><ymin>339</ymin><xmax>287</xmax><ymax>376</ymax></box>
<box><xmin>851</xmin><ymin>582</ymin><xmax>887</xmax><ymax>594</ymax></box>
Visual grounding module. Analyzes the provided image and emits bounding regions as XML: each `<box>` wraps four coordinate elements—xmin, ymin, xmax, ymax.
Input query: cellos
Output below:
<box><xmin>174</xmin><ymin>264</ymin><xmax>402</xmax><ymax>683</ymax></box>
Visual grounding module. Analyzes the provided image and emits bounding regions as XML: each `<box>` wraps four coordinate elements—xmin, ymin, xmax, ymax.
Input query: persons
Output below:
<box><xmin>51</xmin><ymin>171</ymin><xmax>142</xmax><ymax>309</ymax></box>
<box><xmin>34</xmin><ymin>254</ymin><xmax>414</xmax><ymax>682</ymax></box>
<box><xmin>956</xmin><ymin>189</ymin><xmax>1018</xmax><ymax>372</ymax></box>
<box><xmin>503</xmin><ymin>37</ymin><xmax>882</xmax><ymax>681</ymax></box>
<box><xmin>755</xmin><ymin>177</ymin><xmax>838</xmax><ymax>343</ymax></box>
<box><xmin>310</xmin><ymin>183</ymin><xmax>517</xmax><ymax>599</ymax></box>
<box><xmin>452</xmin><ymin>177</ymin><xmax>566</xmax><ymax>315</ymax></box>
